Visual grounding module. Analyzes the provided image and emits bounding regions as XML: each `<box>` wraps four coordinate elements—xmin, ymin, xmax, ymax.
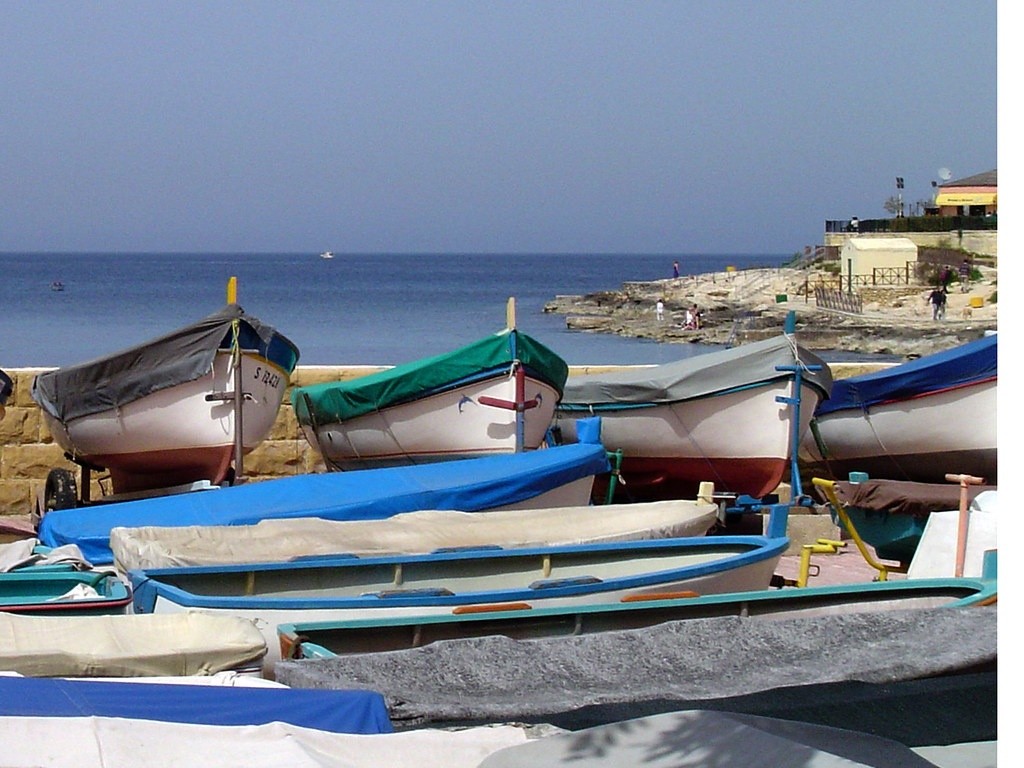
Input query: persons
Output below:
<box><xmin>958</xmin><ymin>259</ymin><xmax>970</xmax><ymax>293</ymax></box>
<box><xmin>939</xmin><ymin>265</ymin><xmax>952</xmax><ymax>295</ymax></box>
<box><xmin>679</xmin><ymin>304</ymin><xmax>702</xmax><ymax>331</ymax></box>
<box><xmin>672</xmin><ymin>261</ymin><xmax>679</xmax><ymax>281</ymax></box>
<box><xmin>985</xmin><ymin>210</ymin><xmax>997</xmax><ymax>230</ymax></box>
<box><xmin>926</xmin><ymin>286</ymin><xmax>947</xmax><ymax>322</ymax></box>
<box><xmin>656</xmin><ymin>299</ymin><xmax>666</xmax><ymax>322</ymax></box>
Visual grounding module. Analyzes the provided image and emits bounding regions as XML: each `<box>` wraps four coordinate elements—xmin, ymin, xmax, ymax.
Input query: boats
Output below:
<box><xmin>811</xmin><ymin>470</ymin><xmax>997</xmax><ymax>593</ymax></box>
<box><xmin>49</xmin><ymin>281</ymin><xmax>64</xmax><ymax>291</ymax></box>
<box><xmin>0</xmin><ymin>531</ymin><xmax>938</xmax><ymax>768</ymax></box>
<box><xmin>778</xmin><ymin>335</ymin><xmax>997</xmax><ymax>490</ymax></box>
<box><xmin>291</xmin><ymin>295</ymin><xmax>569</xmax><ymax>458</ymax></box>
<box><xmin>33</xmin><ymin>414</ymin><xmax>607</xmax><ymax>563</ymax></box>
<box><xmin>320</xmin><ymin>250</ymin><xmax>333</xmax><ymax>258</ymax></box>
<box><xmin>553</xmin><ymin>309</ymin><xmax>835</xmax><ymax>497</ymax></box>
<box><xmin>106</xmin><ymin>481</ymin><xmax>792</xmax><ymax>633</ymax></box>
<box><xmin>31</xmin><ymin>277</ymin><xmax>303</xmax><ymax>487</ymax></box>
<box><xmin>278</xmin><ymin>550</ymin><xmax>998</xmax><ymax>735</ymax></box>
